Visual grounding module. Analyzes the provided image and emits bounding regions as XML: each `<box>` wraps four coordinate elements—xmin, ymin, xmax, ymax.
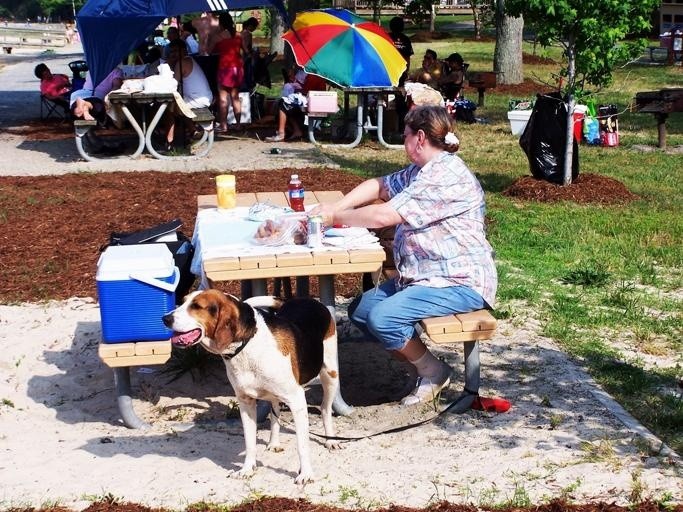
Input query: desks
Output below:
<box><xmin>196</xmin><ymin>189</ymin><xmax>388</xmax><ymax>440</ymax></box>
<box><xmin>108</xmin><ymin>85</ymin><xmax>175</xmax><ymax>164</ymax></box>
<box><xmin>342</xmin><ymin>87</ymin><xmax>402</xmax><ymax>150</ymax></box>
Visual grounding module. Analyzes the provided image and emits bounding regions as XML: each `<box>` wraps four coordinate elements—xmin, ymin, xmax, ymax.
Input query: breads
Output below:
<box><xmin>293</xmin><ymin>226</ymin><xmax>309</xmax><ymax>244</ymax></box>
<box><xmin>254</xmin><ymin>220</ymin><xmax>282</xmax><ymax>238</ymax></box>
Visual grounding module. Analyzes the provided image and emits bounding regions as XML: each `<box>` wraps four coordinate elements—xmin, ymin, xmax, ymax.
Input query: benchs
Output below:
<box><xmin>180</xmin><ymin>107</ymin><xmax>217</xmax><ymax>161</ymax></box>
<box><xmin>363</xmin><ymin>239</ymin><xmax>498</xmax><ymax>416</ymax></box>
<box><xmin>74</xmin><ymin>120</ymin><xmax>98</xmax><ymax>162</ymax></box>
<box><xmin>96</xmin><ymin>336</ymin><xmax>174</xmax><ymax>433</ymax></box>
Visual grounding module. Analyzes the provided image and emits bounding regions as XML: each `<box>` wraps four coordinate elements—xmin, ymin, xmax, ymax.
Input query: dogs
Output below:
<box><xmin>161</xmin><ymin>288</ymin><xmax>357</xmax><ymax>485</ymax></box>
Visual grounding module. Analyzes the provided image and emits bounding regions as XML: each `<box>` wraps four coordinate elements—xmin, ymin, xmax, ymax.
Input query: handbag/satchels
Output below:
<box><xmin>109</xmin><ymin>220</ymin><xmax>195</xmax><ymax>305</ymax></box>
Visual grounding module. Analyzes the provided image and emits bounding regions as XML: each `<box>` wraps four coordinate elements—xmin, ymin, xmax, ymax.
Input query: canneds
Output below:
<box><xmin>306</xmin><ymin>215</ymin><xmax>324</xmax><ymax>248</ymax></box>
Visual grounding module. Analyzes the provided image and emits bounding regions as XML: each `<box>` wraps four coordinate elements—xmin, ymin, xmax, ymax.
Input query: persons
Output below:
<box><xmin>387</xmin><ymin>17</ymin><xmax>464</xmax><ymax>140</ymax></box>
<box><xmin>34</xmin><ymin>11</ymin><xmax>259</xmax><ymax>153</ymax></box>
<box><xmin>307</xmin><ymin>104</ymin><xmax>500</xmax><ymax>409</ymax></box>
<box><xmin>264</xmin><ymin>74</ymin><xmax>326</xmax><ymax>142</ymax></box>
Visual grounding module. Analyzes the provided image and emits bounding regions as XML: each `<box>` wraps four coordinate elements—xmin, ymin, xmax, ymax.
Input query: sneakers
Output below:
<box><xmin>287</xmin><ymin>133</ymin><xmax>301</xmax><ymax>140</ymax></box>
<box><xmin>401</xmin><ymin>362</ymin><xmax>453</xmax><ymax>405</ymax></box>
<box><xmin>214</xmin><ymin>123</ymin><xmax>242</xmax><ymax>132</ymax></box>
<box><xmin>266</xmin><ymin>134</ymin><xmax>284</xmax><ymax>141</ymax></box>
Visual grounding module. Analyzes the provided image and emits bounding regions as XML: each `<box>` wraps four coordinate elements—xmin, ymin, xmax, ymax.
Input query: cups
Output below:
<box><xmin>215</xmin><ymin>174</ymin><xmax>236</xmax><ymax>209</ymax></box>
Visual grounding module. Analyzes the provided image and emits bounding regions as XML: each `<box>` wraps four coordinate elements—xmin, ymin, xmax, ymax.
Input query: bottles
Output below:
<box><xmin>288</xmin><ymin>174</ymin><xmax>305</xmax><ymax>212</ymax></box>
